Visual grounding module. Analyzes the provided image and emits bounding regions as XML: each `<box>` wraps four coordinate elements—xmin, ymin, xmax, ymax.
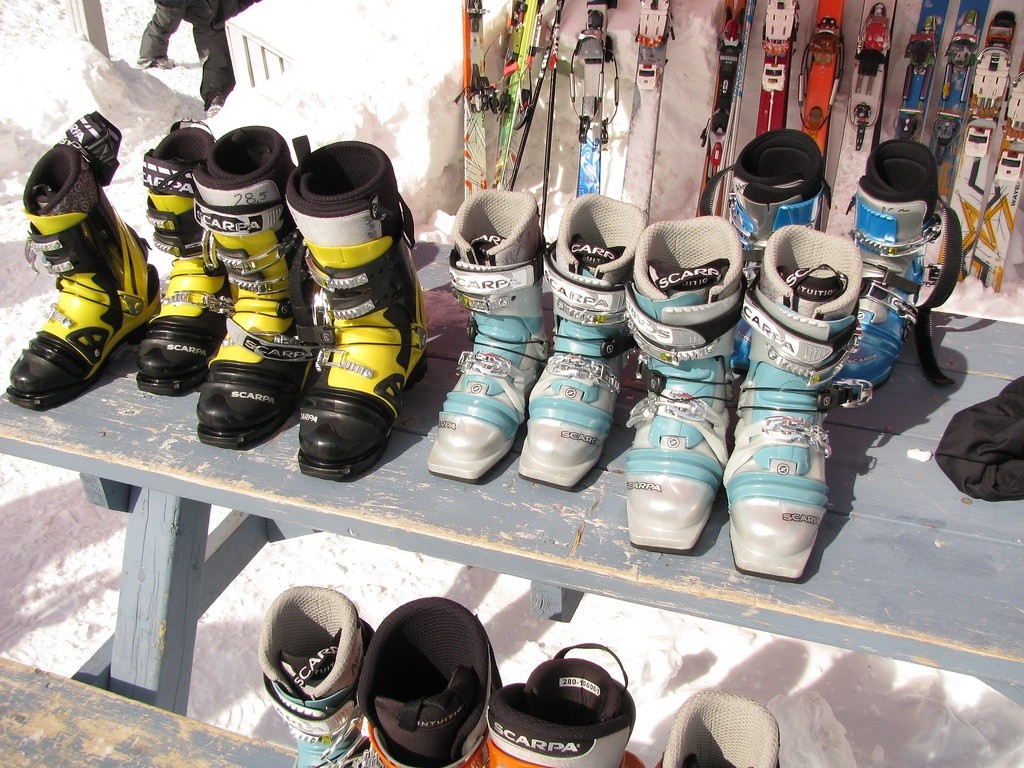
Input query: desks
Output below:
<box><xmin>0</xmin><ymin>241</ymin><xmax>1024</xmax><ymax>718</ymax></box>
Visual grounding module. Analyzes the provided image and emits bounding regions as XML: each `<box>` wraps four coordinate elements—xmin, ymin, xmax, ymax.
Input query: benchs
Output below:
<box><xmin>0</xmin><ymin>654</ymin><xmax>305</xmax><ymax>767</ymax></box>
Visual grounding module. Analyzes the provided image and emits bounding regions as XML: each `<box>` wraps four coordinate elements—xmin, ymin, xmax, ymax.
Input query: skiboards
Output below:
<box><xmin>461</xmin><ymin>1</ymin><xmax>1024</xmax><ymax>295</ymax></box>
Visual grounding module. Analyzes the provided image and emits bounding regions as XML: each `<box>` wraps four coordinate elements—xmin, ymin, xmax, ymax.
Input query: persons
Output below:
<box><xmin>137</xmin><ymin>0</ymin><xmax>260</xmax><ymax>118</ymax></box>
